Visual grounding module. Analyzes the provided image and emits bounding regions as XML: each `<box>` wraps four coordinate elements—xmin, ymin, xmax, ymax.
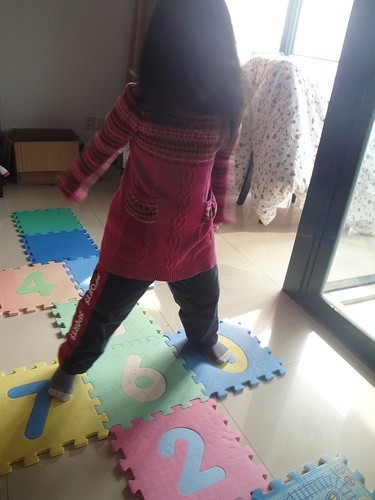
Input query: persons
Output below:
<box><xmin>49</xmin><ymin>0</ymin><xmax>244</xmax><ymax>403</ymax></box>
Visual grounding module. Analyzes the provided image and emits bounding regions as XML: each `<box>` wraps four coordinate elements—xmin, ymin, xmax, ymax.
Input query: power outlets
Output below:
<box><xmin>84</xmin><ymin>117</ymin><xmax>96</xmax><ymax>129</ymax></box>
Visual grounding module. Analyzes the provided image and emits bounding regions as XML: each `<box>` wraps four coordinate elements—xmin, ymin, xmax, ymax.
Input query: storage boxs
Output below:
<box><xmin>0</xmin><ymin>128</ymin><xmax>85</xmax><ymax>186</ymax></box>
